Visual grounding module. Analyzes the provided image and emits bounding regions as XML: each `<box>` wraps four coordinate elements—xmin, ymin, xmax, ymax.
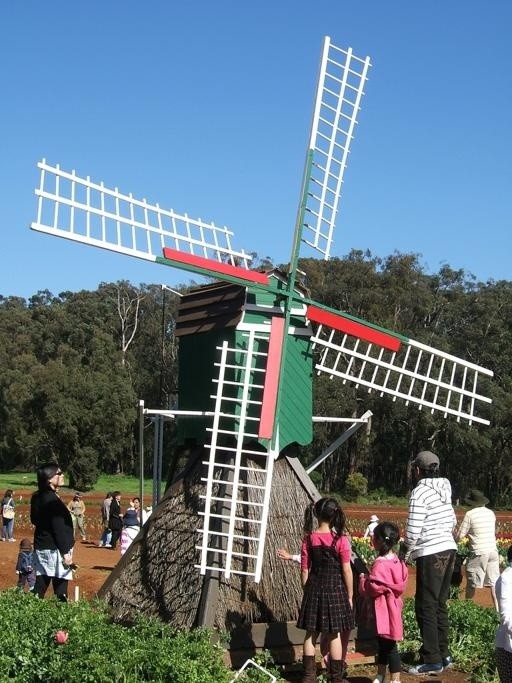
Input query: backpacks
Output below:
<box><xmin>20</xmin><ymin>551</ymin><xmax>34</xmax><ymax>574</ymax></box>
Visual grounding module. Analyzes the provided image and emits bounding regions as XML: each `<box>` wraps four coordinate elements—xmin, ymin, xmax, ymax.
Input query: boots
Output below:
<box><xmin>327</xmin><ymin>654</ymin><xmax>350</xmax><ymax>683</ymax></box>
<box><xmin>301</xmin><ymin>654</ymin><xmax>319</xmax><ymax>683</ymax></box>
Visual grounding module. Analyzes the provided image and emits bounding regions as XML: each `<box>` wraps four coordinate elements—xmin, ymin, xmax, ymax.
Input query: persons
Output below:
<box><xmin>359</xmin><ymin>521</ymin><xmax>409</xmax><ymax>683</ymax></box>
<box><xmin>363</xmin><ymin>514</ymin><xmax>379</xmax><ymax>539</ymax></box>
<box><xmin>275</xmin><ymin>532</ymin><xmax>370</xmax><ymax>673</ymax></box>
<box><xmin>454</xmin><ymin>489</ymin><xmax>503</xmax><ymax>613</ymax></box>
<box><xmin>2</xmin><ymin>462</ymin><xmax>154</xmax><ymax>603</ymax></box>
<box><xmin>299</xmin><ymin>498</ymin><xmax>355</xmax><ymax>682</ymax></box>
<box><xmin>491</xmin><ymin>543</ymin><xmax>511</xmax><ymax>681</ymax></box>
<box><xmin>402</xmin><ymin>450</ymin><xmax>458</xmax><ymax>675</ymax></box>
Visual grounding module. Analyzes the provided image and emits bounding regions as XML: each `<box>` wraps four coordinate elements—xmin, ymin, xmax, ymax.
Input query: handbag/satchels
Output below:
<box><xmin>3</xmin><ymin>497</ymin><xmax>15</xmax><ymax>519</ymax></box>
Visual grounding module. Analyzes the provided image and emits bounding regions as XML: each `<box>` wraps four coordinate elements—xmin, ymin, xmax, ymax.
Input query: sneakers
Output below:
<box><xmin>0</xmin><ymin>537</ymin><xmax>5</xmax><ymax>542</ymax></box>
<box><xmin>408</xmin><ymin>662</ymin><xmax>444</xmax><ymax>676</ymax></box>
<box><xmin>8</xmin><ymin>538</ymin><xmax>17</xmax><ymax>543</ymax></box>
<box><xmin>443</xmin><ymin>656</ymin><xmax>452</xmax><ymax>670</ymax></box>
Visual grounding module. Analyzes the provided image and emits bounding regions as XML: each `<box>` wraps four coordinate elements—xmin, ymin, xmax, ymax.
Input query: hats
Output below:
<box><xmin>410</xmin><ymin>450</ymin><xmax>440</xmax><ymax>471</ymax></box>
<box><xmin>19</xmin><ymin>538</ymin><xmax>32</xmax><ymax>549</ymax></box>
<box><xmin>369</xmin><ymin>514</ymin><xmax>379</xmax><ymax>522</ymax></box>
<box><xmin>122</xmin><ymin>510</ymin><xmax>140</xmax><ymax>526</ymax></box>
<box><xmin>464</xmin><ymin>489</ymin><xmax>490</xmax><ymax>506</ymax></box>
